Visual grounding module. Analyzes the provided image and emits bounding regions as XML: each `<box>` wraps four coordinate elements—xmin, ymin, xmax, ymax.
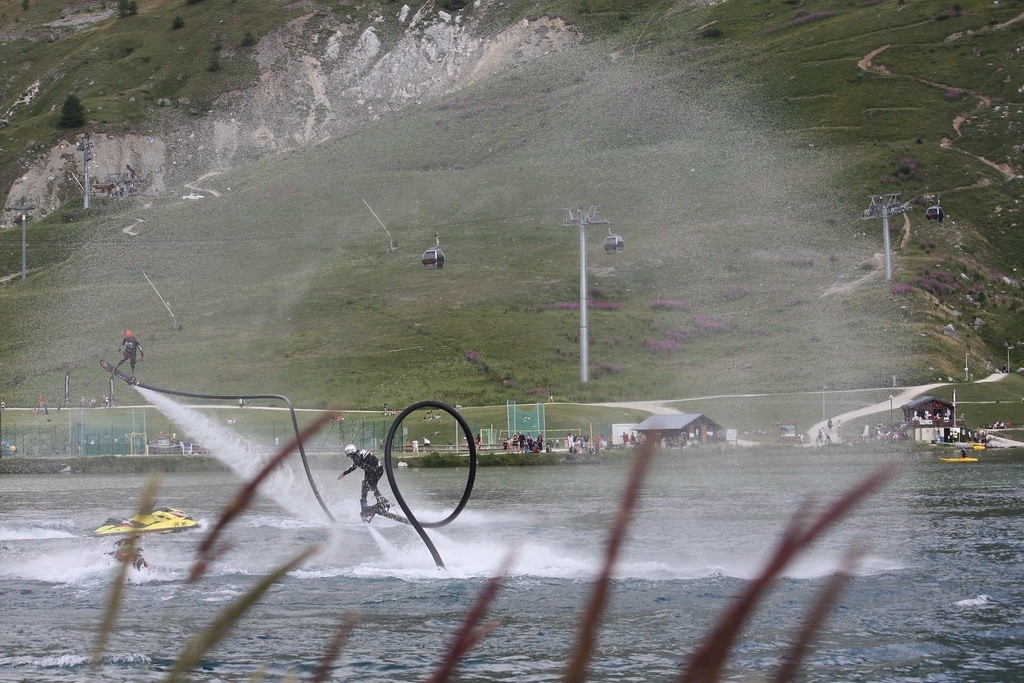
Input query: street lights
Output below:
<box><xmin>965</xmin><ymin>353</ymin><xmax>969</xmax><ymax>380</ymax></box>
<box><xmin>888</xmin><ymin>395</ymin><xmax>892</xmax><ymax>422</ymax></box>
<box><xmin>822</xmin><ymin>385</ymin><xmax>828</xmax><ymax>420</ymax></box>
<box><xmin>1007</xmin><ymin>346</ymin><xmax>1011</xmax><ymax>373</ymax></box>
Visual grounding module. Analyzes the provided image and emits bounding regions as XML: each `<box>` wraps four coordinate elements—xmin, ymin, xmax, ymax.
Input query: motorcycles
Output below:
<box><xmin>87</xmin><ymin>502</ymin><xmax>201</xmax><ymax>537</ymax></box>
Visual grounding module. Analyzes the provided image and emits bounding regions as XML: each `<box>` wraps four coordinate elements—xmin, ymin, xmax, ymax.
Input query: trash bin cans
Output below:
<box><xmin>546</xmin><ymin>443</ymin><xmax>551</xmax><ymax>453</ymax></box>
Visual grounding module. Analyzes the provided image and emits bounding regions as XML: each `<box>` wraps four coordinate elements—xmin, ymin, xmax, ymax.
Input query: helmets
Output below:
<box><xmin>125</xmin><ymin>331</ymin><xmax>131</xmax><ymax>338</ymax></box>
<box><xmin>345</xmin><ymin>444</ymin><xmax>357</xmax><ymax>454</ymax></box>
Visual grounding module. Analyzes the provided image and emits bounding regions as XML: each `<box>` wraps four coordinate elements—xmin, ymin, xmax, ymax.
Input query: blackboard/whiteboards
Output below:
<box><xmin>727</xmin><ymin>429</ymin><xmax>737</xmax><ymax>441</ymax></box>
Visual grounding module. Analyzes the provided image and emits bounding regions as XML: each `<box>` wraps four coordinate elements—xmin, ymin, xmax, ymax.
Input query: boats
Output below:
<box><xmin>938</xmin><ymin>456</ymin><xmax>977</xmax><ymax>462</ymax></box>
<box><xmin>972</xmin><ymin>444</ymin><xmax>986</xmax><ymax>451</ymax></box>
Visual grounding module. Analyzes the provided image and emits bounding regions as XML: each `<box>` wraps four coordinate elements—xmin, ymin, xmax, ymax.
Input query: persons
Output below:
<box><xmin>816</xmin><ymin>417</ymin><xmax>1014</xmax><ymax>445</ymax></box>
<box><xmin>79</xmin><ymin>394</ymin><xmax>109</xmax><ymax>411</ymax></box>
<box><xmin>240</xmin><ymin>397</ymin><xmax>252</xmax><ymax>409</ymax></box>
<box><xmin>961</xmin><ymin>449</ymin><xmax>967</xmax><ymax>458</ymax></box>
<box><xmin>506</xmin><ymin>431</ymin><xmax>543</xmax><ymax>459</ymax></box>
<box><xmin>475</xmin><ymin>433</ymin><xmax>481</xmax><ymax>450</ymax></box>
<box><xmin>38</xmin><ymin>395</ymin><xmax>44</xmax><ymax>408</ymax></box>
<box><xmin>551</xmin><ymin>429</ymin><xmax>688</xmax><ymax>456</ymax></box>
<box><xmin>422</xmin><ymin>410</ymin><xmax>441</xmax><ymax>421</ymax></box>
<box><xmin>548</xmin><ymin>391</ymin><xmax>554</xmax><ymax>403</ymax></box>
<box><xmin>113</xmin><ymin>330</ymin><xmax>144</xmax><ymax>382</ymax></box>
<box><xmin>410</xmin><ymin>435</ymin><xmax>431</xmax><ymax>453</ymax></box>
<box><xmin>336</xmin><ymin>443</ymin><xmax>388</xmax><ymax>510</ymax></box>
